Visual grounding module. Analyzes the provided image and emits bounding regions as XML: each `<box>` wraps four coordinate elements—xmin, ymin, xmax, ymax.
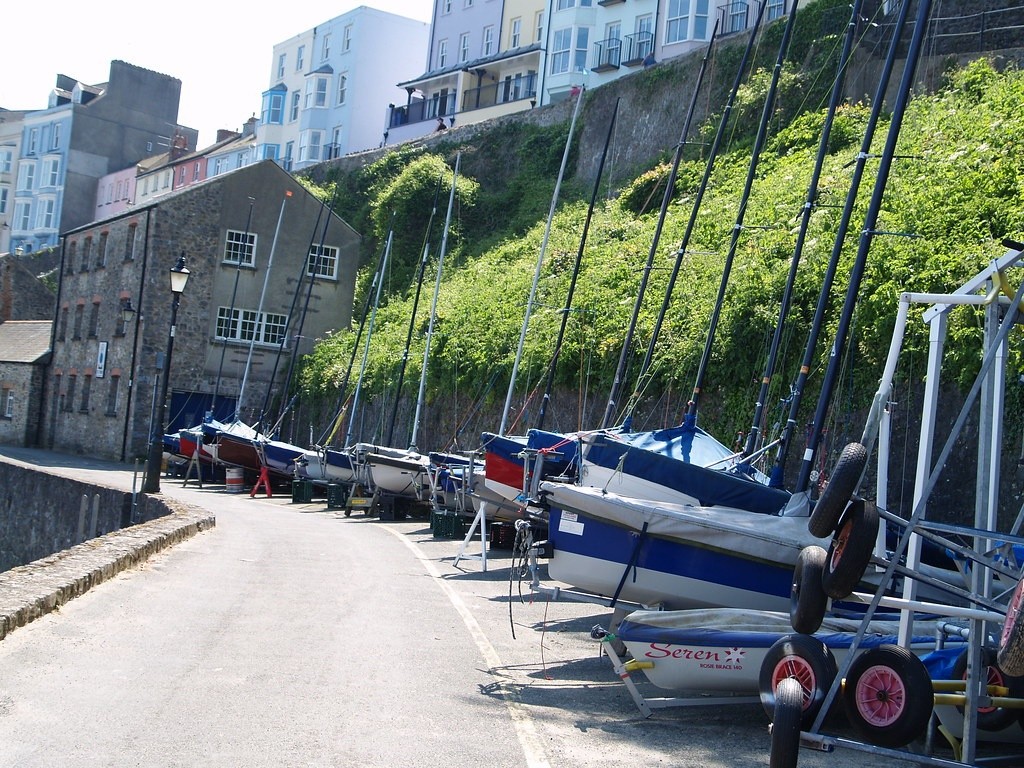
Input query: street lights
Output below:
<box><xmin>140</xmin><ymin>249</ymin><xmax>190</xmax><ymax>493</ymax></box>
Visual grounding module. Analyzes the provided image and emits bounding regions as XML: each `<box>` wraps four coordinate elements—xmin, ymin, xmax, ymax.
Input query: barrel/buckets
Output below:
<box><xmin>226</xmin><ymin>468</ymin><xmax>244</xmax><ymax>493</ymax></box>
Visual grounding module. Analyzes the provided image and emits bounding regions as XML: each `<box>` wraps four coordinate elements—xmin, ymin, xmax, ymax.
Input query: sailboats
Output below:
<box><xmin>162</xmin><ymin>0</ymin><xmax>1022</xmax><ymax>752</ymax></box>
